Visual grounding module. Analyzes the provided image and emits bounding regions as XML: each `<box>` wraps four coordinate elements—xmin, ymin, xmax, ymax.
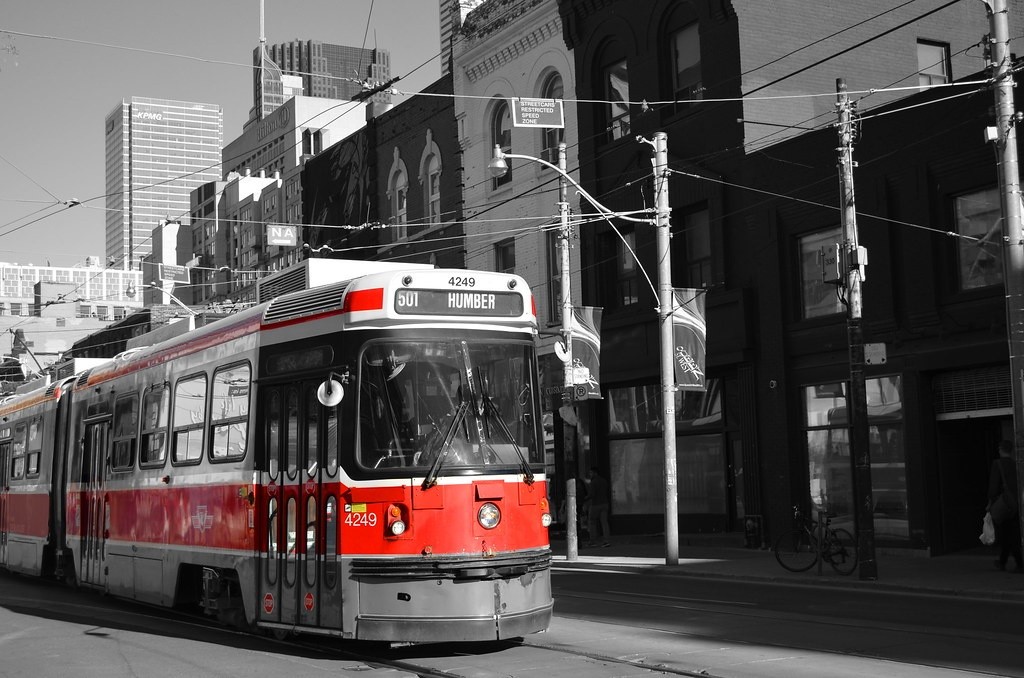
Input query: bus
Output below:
<box><xmin>822</xmin><ymin>402</ymin><xmax>909</xmax><ymax>517</ymax></box>
<box><xmin>1</xmin><ymin>255</ymin><xmax>556</xmax><ymax>651</ymax></box>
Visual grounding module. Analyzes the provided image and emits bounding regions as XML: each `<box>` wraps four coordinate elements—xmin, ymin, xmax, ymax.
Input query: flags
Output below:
<box><xmin>672</xmin><ymin>287</ymin><xmax>707</xmax><ymax>391</ymax></box>
<box><xmin>570</xmin><ymin>307</ymin><xmax>603</xmax><ymax>398</ymax></box>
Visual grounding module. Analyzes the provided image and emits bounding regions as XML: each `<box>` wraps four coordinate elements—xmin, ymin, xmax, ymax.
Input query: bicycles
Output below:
<box><xmin>774</xmin><ymin>504</ymin><xmax>858</xmax><ymax>577</ymax></box>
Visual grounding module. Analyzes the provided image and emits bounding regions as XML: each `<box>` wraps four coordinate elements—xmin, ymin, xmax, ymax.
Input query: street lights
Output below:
<box><xmin>485</xmin><ymin>129</ymin><xmax>681</xmax><ymax>568</ymax></box>
